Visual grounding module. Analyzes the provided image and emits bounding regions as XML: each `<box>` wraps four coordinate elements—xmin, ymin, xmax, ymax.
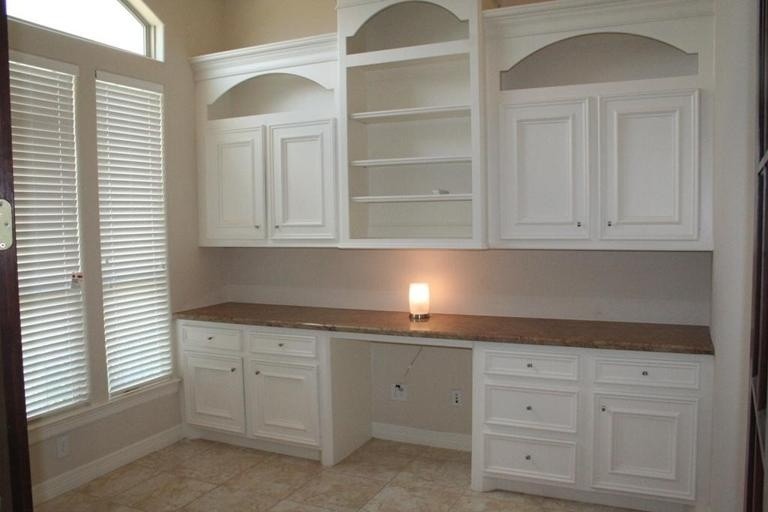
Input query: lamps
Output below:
<box><xmin>408</xmin><ymin>282</ymin><xmax>431</xmax><ymax>320</ymax></box>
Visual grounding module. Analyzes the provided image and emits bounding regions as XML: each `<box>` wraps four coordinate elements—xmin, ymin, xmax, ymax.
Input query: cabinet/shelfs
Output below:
<box><xmin>176</xmin><ymin>319</ymin><xmax>373</xmax><ymax>467</ymax></box>
<box><xmin>483</xmin><ymin>0</ymin><xmax>714</xmax><ymax>252</ymax></box>
<box><xmin>472</xmin><ymin>341</ymin><xmax>715</xmax><ymax>512</ymax></box>
<box><xmin>335</xmin><ymin>1</ymin><xmax>484</xmax><ymax>249</ymax></box>
<box><xmin>186</xmin><ymin>33</ymin><xmax>338</xmax><ymax>247</ymax></box>
